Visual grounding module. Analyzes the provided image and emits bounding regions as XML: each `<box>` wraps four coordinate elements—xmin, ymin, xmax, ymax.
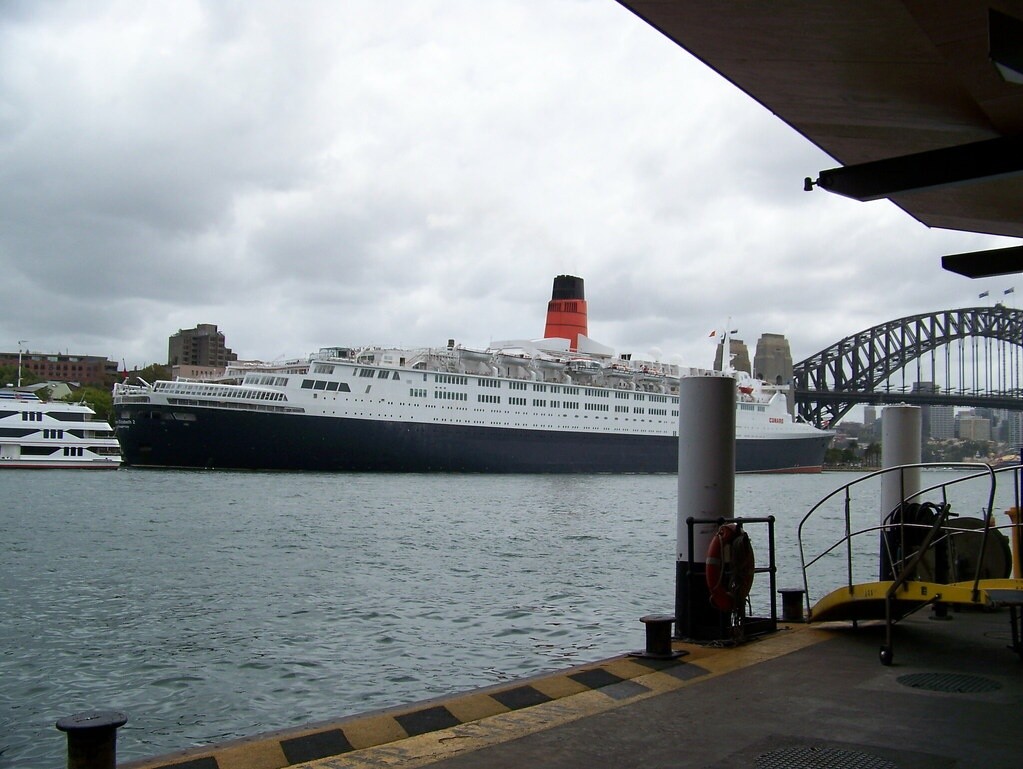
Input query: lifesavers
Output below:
<box><xmin>702</xmin><ymin>523</ymin><xmax>757</xmax><ymax>611</ymax></box>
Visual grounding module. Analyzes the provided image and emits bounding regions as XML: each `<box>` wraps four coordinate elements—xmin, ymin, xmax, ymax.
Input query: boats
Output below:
<box><xmin>107</xmin><ymin>274</ymin><xmax>836</xmax><ymax>473</ymax></box>
<box><xmin>0</xmin><ymin>340</ymin><xmax>125</xmax><ymax>469</ymax></box>
